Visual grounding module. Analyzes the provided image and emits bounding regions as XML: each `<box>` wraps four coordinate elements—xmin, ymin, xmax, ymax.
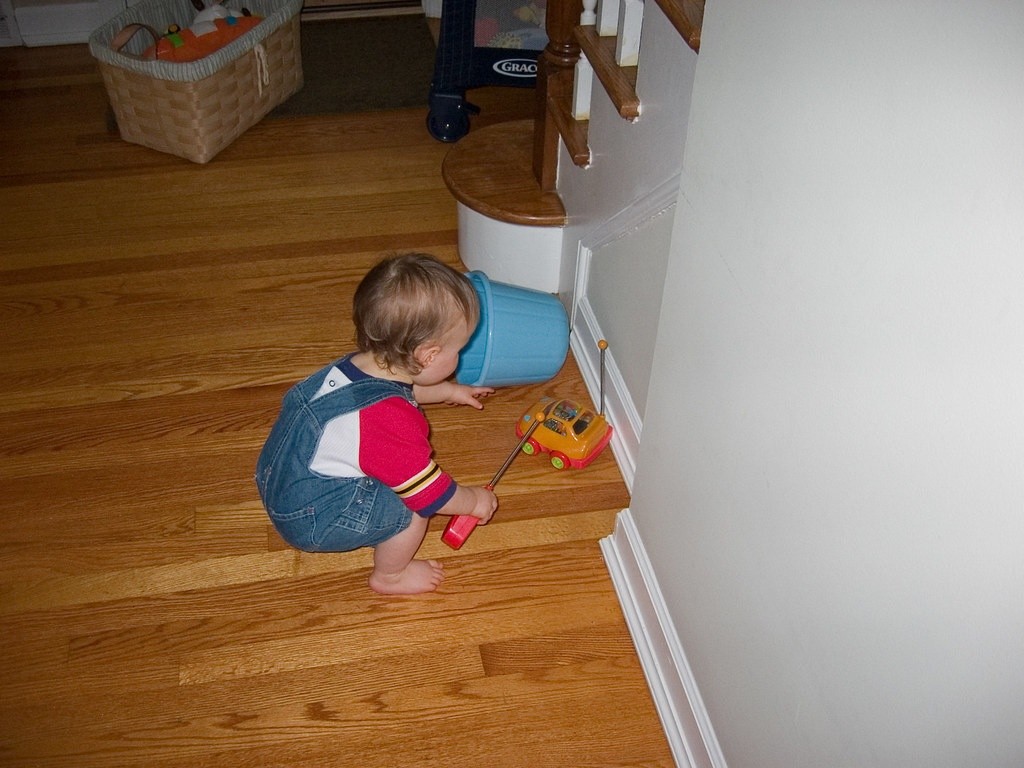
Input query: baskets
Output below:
<box><xmin>90</xmin><ymin>0</ymin><xmax>306</xmax><ymax>164</ymax></box>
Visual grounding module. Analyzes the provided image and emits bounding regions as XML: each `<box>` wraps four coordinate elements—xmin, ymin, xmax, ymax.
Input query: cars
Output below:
<box><xmin>516</xmin><ymin>397</ymin><xmax>612</xmax><ymax>471</ymax></box>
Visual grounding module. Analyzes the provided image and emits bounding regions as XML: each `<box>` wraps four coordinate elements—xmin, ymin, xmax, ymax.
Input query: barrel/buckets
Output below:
<box><xmin>454</xmin><ymin>271</ymin><xmax>569</xmax><ymax>387</ymax></box>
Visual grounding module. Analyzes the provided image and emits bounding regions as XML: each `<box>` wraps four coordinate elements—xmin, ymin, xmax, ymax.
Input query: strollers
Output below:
<box><xmin>425</xmin><ymin>0</ymin><xmax>550</xmax><ymax>142</ymax></box>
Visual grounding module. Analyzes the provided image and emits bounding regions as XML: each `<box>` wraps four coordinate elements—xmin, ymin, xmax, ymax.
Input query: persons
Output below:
<box><xmin>256</xmin><ymin>252</ymin><xmax>498</xmax><ymax>595</ymax></box>
